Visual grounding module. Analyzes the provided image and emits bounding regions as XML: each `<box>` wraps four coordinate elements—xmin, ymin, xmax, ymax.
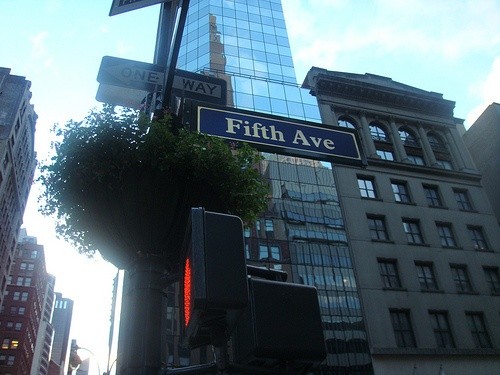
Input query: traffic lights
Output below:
<box><xmin>177</xmin><ymin>205</ymin><xmax>249</xmax><ymax>349</ymax></box>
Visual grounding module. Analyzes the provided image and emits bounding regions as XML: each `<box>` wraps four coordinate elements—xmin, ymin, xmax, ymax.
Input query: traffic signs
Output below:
<box><xmin>95</xmin><ymin>54</ymin><xmax>369</xmax><ymax>168</ymax></box>
